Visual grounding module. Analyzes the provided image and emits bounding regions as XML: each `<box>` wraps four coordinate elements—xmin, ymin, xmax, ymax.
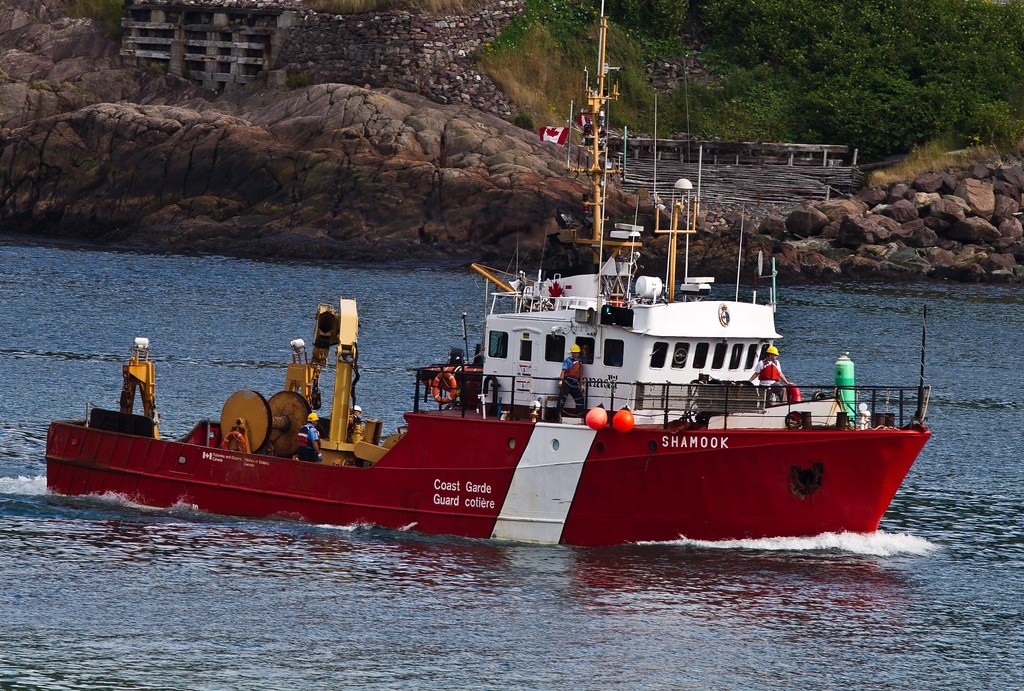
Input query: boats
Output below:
<box><xmin>44</xmin><ymin>0</ymin><xmax>931</xmax><ymax>544</ymax></box>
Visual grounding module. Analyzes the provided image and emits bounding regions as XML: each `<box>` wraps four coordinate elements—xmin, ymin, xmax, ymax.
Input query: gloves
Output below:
<box><xmin>580</xmin><ymin>385</ymin><xmax>585</xmax><ymax>393</ymax></box>
<box><xmin>318</xmin><ymin>453</ymin><xmax>323</xmax><ymax>462</ymax></box>
<box><xmin>558</xmin><ymin>381</ymin><xmax>562</xmax><ymax>388</ymax></box>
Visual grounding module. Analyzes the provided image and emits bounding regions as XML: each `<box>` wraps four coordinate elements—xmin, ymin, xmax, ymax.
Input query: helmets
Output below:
<box><xmin>766</xmin><ymin>346</ymin><xmax>779</xmax><ymax>356</ymax></box>
<box><xmin>306</xmin><ymin>413</ymin><xmax>319</xmax><ymax>422</ymax></box>
<box><xmin>351</xmin><ymin>405</ymin><xmax>362</xmax><ymax>412</ymax></box>
<box><xmin>570</xmin><ymin>344</ymin><xmax>581</xmax><ymax>353</ymax></box>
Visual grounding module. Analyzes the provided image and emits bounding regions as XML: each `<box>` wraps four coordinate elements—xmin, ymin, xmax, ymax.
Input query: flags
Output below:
<box><xmin>539</xmin><ymin>126</ymin><xmax>570</xmax><ymax>145</ymax></box>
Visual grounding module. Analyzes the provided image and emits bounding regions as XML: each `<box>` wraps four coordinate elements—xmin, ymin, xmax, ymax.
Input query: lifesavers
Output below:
<box><xmin>432</xmin><ymin>372</ymin><xmax>457</xmax><ymax>404</ymax></box>
<box><xmin>785</xmin><ymin>383</ymin><xmax>802</xmax><ymax>404</ymax></box>
<box><xmin>223</xmin><ymin>431</ymin><xmax>246</xmax><ymax>453</ymax></box>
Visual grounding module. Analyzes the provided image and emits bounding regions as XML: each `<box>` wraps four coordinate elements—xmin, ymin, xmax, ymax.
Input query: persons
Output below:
<box><xmin>748</xmin><ymin>346</ymin><xmax>795</xmax><ymax>408</ymax></box>
<box><xmin>347</xmin><ymin>405</ymin><xmax>365</xmax><ymax>468</ymax></box>
<box><xmin>297</xmin><ymin>412</ymin><xmax>323</xmax><ymax>463</ymax></box>
<box><xmin>580</xmin><ymin>344</ymin><xmax>593</xmax><ymax>364</ymax></box>
<box><xmin>556</xmin><ymin>344</ymin><xmax>585</xmax><ymax>424</ymax></box>
